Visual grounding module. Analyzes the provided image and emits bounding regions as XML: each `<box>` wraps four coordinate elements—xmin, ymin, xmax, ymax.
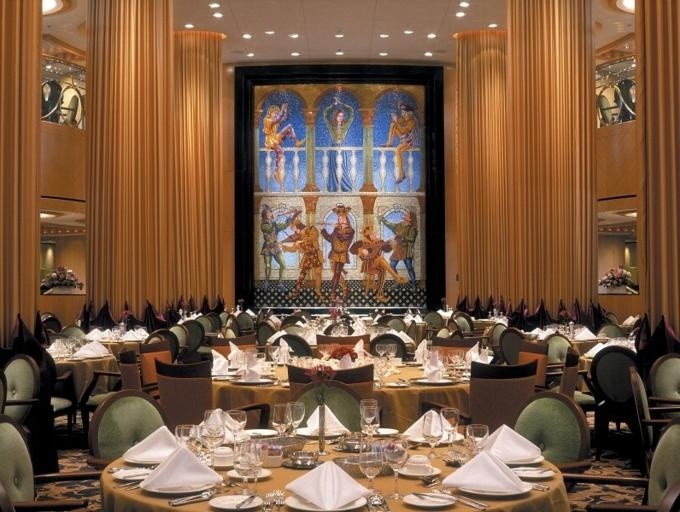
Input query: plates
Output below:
<box><xmin>458</xmin><ymin>481</ymin><xmax>533</xmax><ymax>496</ymax></box>
<box><xmin>207</xmin><ymin>463</ymin><xmax>234</xmax><ymax>469</ymax></box>
<box><xmin>228</xmin><ymin>366</ymin><xmax>240</xmax><ymax>370</ymax></box>
<box><xmin>332</xmin><ymin>445</ymin><xmax>368</xmax><ymax>452</ymax></box>
<box><xmin>393</xmin><ymin>364</ymin><xmax>406</xmax><ymax>367</ymax></box>
<box><xmin>283</xmin><ymin>460</ymin><xmax>324</xmax><ymax>470</ymax></box>
<box><xmin>375</xmin><ymin>382</ymin><xmax>410</xmax><ymax>387</ymax></box>
<box><xmin>245</xmin><ymin>428</ymin><xmax>278</xmax><ymax>437</ymax></box>
<box><xmin>418</xmin><ymin>367</ymin><xmax>426</xmax><ymax>370</ymax></box>
<box><xmin>227</xmin><ymin>468</ymin><xmax>272</xmax><ymax>479</ymax></box>
<box><xmin>409</xmin><ymin>435</ymin><xmax>464</xmax><ymax>446</ymax></box>
<box><xmin>505</xmin><ymin>456</ymin><xmax>544</xmax><ymax>465</ymax></box>
<box><xmin>211</xmin><ymin>370</ymin><xmax>236</xmax><ymax>376</ymax></box>
<box><xmin>285</xmin><ymin>494</ymin><xmax>367</xmax><ymax>512</ymax></box>
<box><xmin>73</xmin><ymin>352</ymin><xmax>113</xmax><ymax>359</ymax></box>
<box><xmin>398</xmin><ymin>467</ymin><xmax>441</xmax><ymax>479</ymax></box>
<box><xmin>293</xmin><ymin>427</ymin><xmax>342</xmax><ymax>439</ymax></box>
<box><xmin>229</xmin><ymin>379</ymin><xmax>273</xmax><ymax>385</ymax></box>
<box><xmin>361</xmin><ymin>428</ymin><xmax>400</xmax><ymax>436</ymax></box>
<box><xmin>413</xmin><ymin>378</ymin><xmax>458</xmax><ymax>385</ymax></box>
<box><xmin>139</xmin><ymin>481</ymin><xmax>217</xmax><ymax>494</ymax></box>
<box><xmin>98</xmin><ymin>340</ymin><xmax>113</xmax><ymax>342</ymax></box>
<box><xmin>406</xmin><ymin>361</ymin><xmax>423</xmax><ymax>365</ymax></box>
<box><xmin>209</xmin><ymin>495</ymin><xmax>263</xmax><ymax>510</ymax></box>
<box><xmin>124</xmin><ymin>338</ymin><xmax>144</xmax><ymax>342</ymax></box>
<box><xmin>402</xmin><ymin>492</ymin><xmax>457</xmax><ymax>508</ymax></box>
<box><xmin>124</xmin><ymin>457</ymin><xmax>161</xmax><ymax>464</ymax></box>
<box><xmin>511</xmin><ymin>467</ymin><xmax>555</xmax><ymax>479</ymax></box>
<box><xmin>111</xmin><ymin>468</ymin><xmax>153</xmax><ymax>481</ymax></box>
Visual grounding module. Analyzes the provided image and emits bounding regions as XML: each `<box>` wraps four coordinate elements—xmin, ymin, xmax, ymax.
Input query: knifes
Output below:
<box><xmin>123</xmin><ymin>472</ymin><xmax>150</xmax><ymax>479</ymax></box>
<box><xmin>383</xmin><ymin>497</ymin><xmax>390</xmax><ymax>512</ymax></box>
<box><xmin>235</xmin><ymin>494</ymin><xmax>259</xmax><ymax>508</ymax></box>
<box><xmin>412</xmin><ymin>491</ymin><xmax>485</xmax><ymax>511</ymax></box>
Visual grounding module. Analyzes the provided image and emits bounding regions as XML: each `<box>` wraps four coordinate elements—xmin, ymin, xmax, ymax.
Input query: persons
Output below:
<box><xmin>261</xmin><ymin>101</ymin><xmax>306</xmax><ymax>184</ymax></box>
<box><xmin>350</xmin><ymin>225</ymin><xmax>408</xmax><ymax>304</ymax></box>
<box><xmin>260</xmin><ymin>202</ymin><xmax>297</xmax><ymax>292</ymax></box>
<box><xmin>323</xmin><ymin>92</ymin><xmax>356</xmax><ymax>193</ymax></box>
<box><xmin>378</xmin><ymin>101</ymin><xmax>419</xmax><ymax>184</ymax></box>
<box><xmin>279</xmin><ymin>210</ymin><xmax>324</xmax><ymax>298</ymax></box>
<box><xmin>318</xmin><ymin>203</ymin><xmax>356</xmax><ymax>303</ymax></box>
<box><xmin>372</xmin><ymin>206</ymin><xmax>418</xmax><ymax>293</ymax></box>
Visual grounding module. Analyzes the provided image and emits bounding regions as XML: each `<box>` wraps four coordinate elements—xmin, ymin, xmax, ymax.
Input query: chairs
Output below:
<box><xmin>39</xmin><ymin>77</ymin><xmax>81</xmax><ymax>127</ymax></box>
<box><xmin>598</xmin><ymin>76</ymin><xmax>637</xmax><ymax>126</ymax></box>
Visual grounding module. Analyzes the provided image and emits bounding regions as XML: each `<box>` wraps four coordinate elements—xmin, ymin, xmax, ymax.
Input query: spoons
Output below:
<box><xmin>368</xmin><ymin>494</ymin><xmax>384</xmax><ymax>511</ymax></box>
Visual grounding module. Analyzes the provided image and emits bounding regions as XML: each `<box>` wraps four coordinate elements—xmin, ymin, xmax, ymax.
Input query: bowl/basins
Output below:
<box><xmin>339</xmin><ymin>432</ymin><xmax>367</xmax><ymax>448</ymax></box>
<box><xmin>261</xmin><ymin>436</ymin><xmax>307</xmax><ymax>456</ymax></box>
<box><xmin>333</xmin><ymin>455</ymin><xmax>410</xmax><ymax>477</ymax></box>
<box><xmin>289</xmin><ymin>451</ymin><xmax>319</xmax><ymax>465</ymax></box>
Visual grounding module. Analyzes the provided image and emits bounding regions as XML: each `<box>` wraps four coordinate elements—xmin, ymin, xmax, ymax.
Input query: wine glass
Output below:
<box><xmin>224</xmin><ymin>410</ymin><xmax>247</xmax><ymax>449</ymax></box>
<box><xmin>446</xmin><ymin>349</ymin><xmax>472</xmax><ymax>375</ymax></box>
<box><xmin>247</xmin><ymin>441</ymin><xmax>268</xmax><ymax>493</ymax></box>
<box><xmin>236</xmin><ymin>344</ymin><xmax>281</xmax><ymax>368</ymax></box>
<box><xmin>375</xmin><ymin>344</ymin><xmax>398</xmax><ymax>362</ymax></box>
<box><xmin>272</xmin><ymin>403</ymin><xmax>292</xmax><ymax>435</ymax></box>
<box><xmin>53</xmin><ymin>335</ymin><xmax>88</xmax><ymax>360</ymax></box>
<box><xmin>233</xmin><ymin>440</ymin><xmax>255</xmax><ymax>493</ymax></box>
<box><xmin>175</xmin><ymin>424</ymin><xmax>199</xmax><ymax>448</ymax></box>
<box><xmin>358</xmin><ymin>439</ymin><xmax>383</xmax><ymax>495</ymax></box>
<box><xmin>452</xmin><ymin>425</ymin><xmax>476</xmax><ymax>466</ymax></box>
<box><xmin>440</xmin><ymin>407</ymin><xmax>459</xmax><ymax>454</ymax></box>
<box><xmin>204</xmin><ymin>409</ymin><xmax>224</xmax><ymax>438</ymax></box>
<box><xmin>607</xmin><ymin>336</ymin><xmax>635</xmax><ymax>351</ymax></box>
<box><xmin>359</xmin><ymin>399</ymin><xmax>378</xmax><ymax>425</ymax></box>
<box><xmin>359</xmin><ymin>407</ymin><xmax>380</xmax><ymax>439</ymax></box>
<box><xmin>286</xmin><ymin>402</ymin><xmax>305</xmax><ymax>436</ymax></box>
<box><xmin>467</xmin><ymin>424</ymin><xmax>489</xmax><ymax>452</ymax></box>
<box><xmin>383</xmin><ymin>439</ymin><xmax>409</xmax><ymax>500</ymax></box>
<box><xmin>200</xmin><ymin>424</ymin><xmax>225</xmax><ymax>468</ymax></box>
<box><xmin>423</xmin><ymin>414</ymin><xmax>443</xmax><ymax>459</ymax></box>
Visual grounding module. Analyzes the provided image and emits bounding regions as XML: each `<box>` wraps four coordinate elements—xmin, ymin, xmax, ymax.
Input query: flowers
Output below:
<box><xmin>39</xmin><ymin>264</ymin><xmax>85</xmax><ymax>292</ymax></box>
<box><xmin>600</xmin><ymin>267</ymin><xmax>633</xmax><ymax>287</ymax></box>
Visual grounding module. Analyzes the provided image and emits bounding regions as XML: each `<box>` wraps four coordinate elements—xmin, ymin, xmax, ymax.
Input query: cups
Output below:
<box><xmin>546</xmin><ymin>319</ymin><xmax>576</xmax><ymax>342</ymax></box>
<box><xmin>332</xmin><ymin>326</ymin><xmax>348</xmax><ymax>335</ymax></box>
<box><xmin>214</xmin><ymin>447</ymin><xmax>233</xmax><ymax>465</ymax></box>
<box><xmin>407</xmin><ymin>455</ymin><xmax>433</xmax><ymax>474</ymax></box>
<box><xmin>393</xmin><ymin>358</ymin><xmax>402</xmax><ymax>364</ymax></box>
<box><xmin>112</xmin><ymin>323</ymin><xmax>126</xmax><ymax>339</ymax></box>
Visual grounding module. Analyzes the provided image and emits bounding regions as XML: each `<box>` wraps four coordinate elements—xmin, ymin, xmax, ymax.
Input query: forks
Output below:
<box><xmin>261</xmin><ymin>493</ymin><xmax>273</xmax><ymax>512</ymax></box>
<box><xmin>434</xmin><ymin>487</ymin><xmax>489</xmax><ymax>507</ymax></box>
<box><xmin>273</xmin><ymin>489</ymin><xmax>286</xmax><ymax>512</ymax></box>
